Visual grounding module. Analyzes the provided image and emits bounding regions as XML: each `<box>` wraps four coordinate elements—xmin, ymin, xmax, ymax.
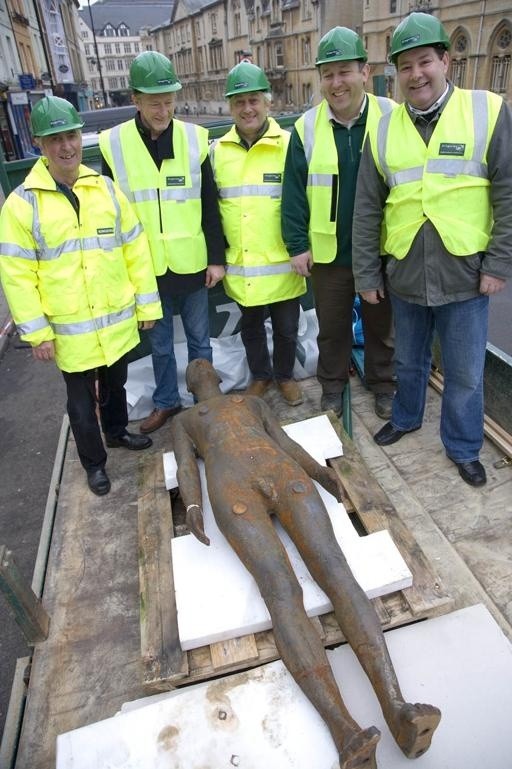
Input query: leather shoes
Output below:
<box><xmin>88</xmin><ymin>469</ymin><xmax>110</xmax><ymax>495</ymax></box>
<box><xmin>106</xmin><ymin>432</ymin><xmax>153</xmax><ymax>450</ymax></box>
<box><xmin>373</xmin><ymin>422</ymin><xmax>421</xmax><ymax>446</ymax></box>
<box><xmin>140</xmin><ymin>405</ymin><xmax>181</xmax><ymax>434</ymax></box>
<box><xmin>446</xmin><ymin>455</ymin><xmax>486</xmax><ymax>486</ymax></box>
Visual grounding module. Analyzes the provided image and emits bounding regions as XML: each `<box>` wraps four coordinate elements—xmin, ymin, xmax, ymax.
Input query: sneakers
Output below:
<box><xmin>375</xmin><ymin>393</ymin><xmax>396</xmax><ymax>419</ymax></box>
<box><xmin>278</xmin><ymin>378</ymin><xmax>302</xmax><ymax>406</ymax></box>
<box><xmin>320</xmin><ymin>392</ymin><xmax>343</xmax><ymax>419</ymax></box>
<box><xmin>244</xmin><ymin>379</ymin><xmax>273</xmax><ymax>398</ymax></box>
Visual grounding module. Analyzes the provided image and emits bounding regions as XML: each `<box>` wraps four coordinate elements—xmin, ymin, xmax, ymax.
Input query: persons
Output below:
<box><xmin>0</xmin><ymin>95</ymin><xmax>165</xmax><ymax>494</ymax></box>
<box><xmin>97</xmin><ymin>50</ymin><xmax>227</xmax><ymax>434</ymax></box>
<box><xmin>351</xmin><ymin>11</ymin><xmax>511</xmax><ymax>488</ymax></box>
<box><xmin>161</xmin><ymin>356</ymin><xmax>442</xmax><ymax>769</ymax></box>
<box><xmin>211</xmin><ymin>60</ymin><xmax>310</xmax><ymax>406</ymax></box>
<box><xmin>279</xmin><ymin>25</ymin><xmax>401</xmax><ymax>419</ymax></box>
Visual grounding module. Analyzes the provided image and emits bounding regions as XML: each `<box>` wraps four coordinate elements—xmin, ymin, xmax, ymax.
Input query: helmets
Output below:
<box><xmin>224</xmin><ymin>62</ymin><xmax>270</xmax><ymax>96</ymax></box>
<box><xmin>314</xmin><ymin>26</ymin><xmax>367</xmax><ymax>64</ymax></box>
<box><xmin>129</xmin><ymin>51</ymin><xmax>183</xmax><ymax>94</ymax></box>
<box><xmin>30</xmin><ymin>96</ymin><xmax>84</xmax><ymax>137</ymax></box>
<box><xmin>388</xmin><ymin>12</ymin><xmax>450</xmax><ymax>58</ymax></box>
